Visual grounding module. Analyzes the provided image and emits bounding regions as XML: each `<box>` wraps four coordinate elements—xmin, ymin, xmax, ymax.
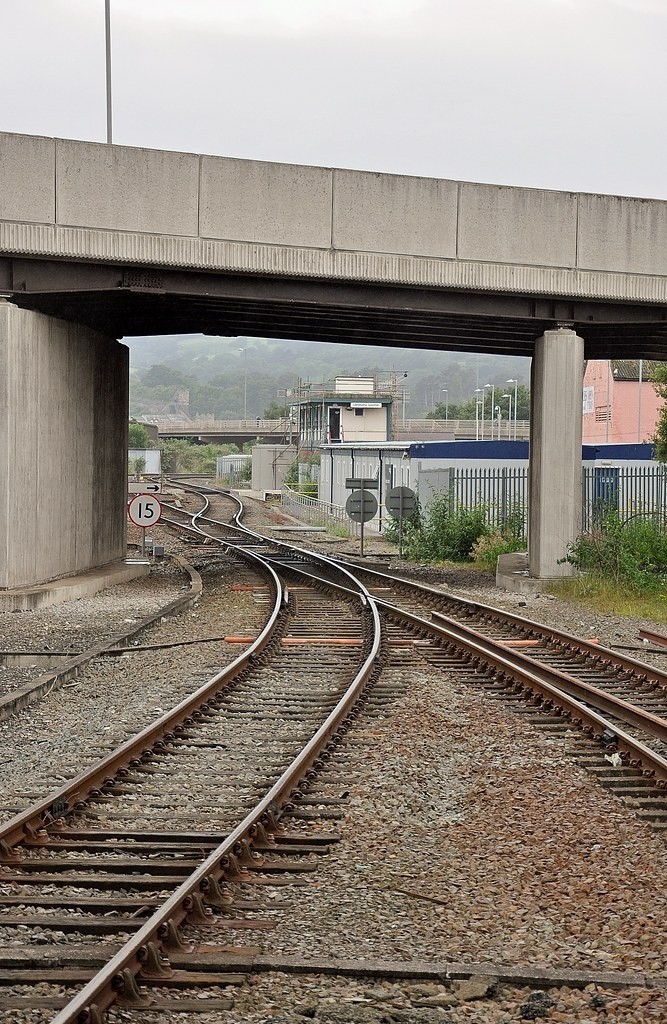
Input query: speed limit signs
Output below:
<box><xmin>127</xmin><ymin>495</ymin><xmax>161</xmax><ymax>527</ymax></box>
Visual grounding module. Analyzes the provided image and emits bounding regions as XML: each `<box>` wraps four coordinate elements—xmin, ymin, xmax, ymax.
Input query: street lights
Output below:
<box><xmin>442</xmin><ymin>390</ymin><xmax>448</xmax><ymax>427</ymax></box>
<box><xmin>475</xmin><ymin>389</ymin><xmax>483</xmax><ymax>440</ymax></box>
<box><xmin>236</xmin><ymin>348</ymin><xmax>247</xmax><ymax>420</ymax></box>
<box><xmin>483</xmin><ymin>383</ymin><xmax>493</xmax><ymax>440</ymax></box>
<box><xmin>476</xmin><ymin>401</ymin><xmax>482</xmax><ymax>440</ymax></box>
<box><xmin>505</xmin><ymin>379</ymin><xmax>517</xmax><ymax>441</ymax></box>
<box><xmin>500</xmin><ymin>395</ymin><xmax>512</xmax><ymax>441</ymax></box>
